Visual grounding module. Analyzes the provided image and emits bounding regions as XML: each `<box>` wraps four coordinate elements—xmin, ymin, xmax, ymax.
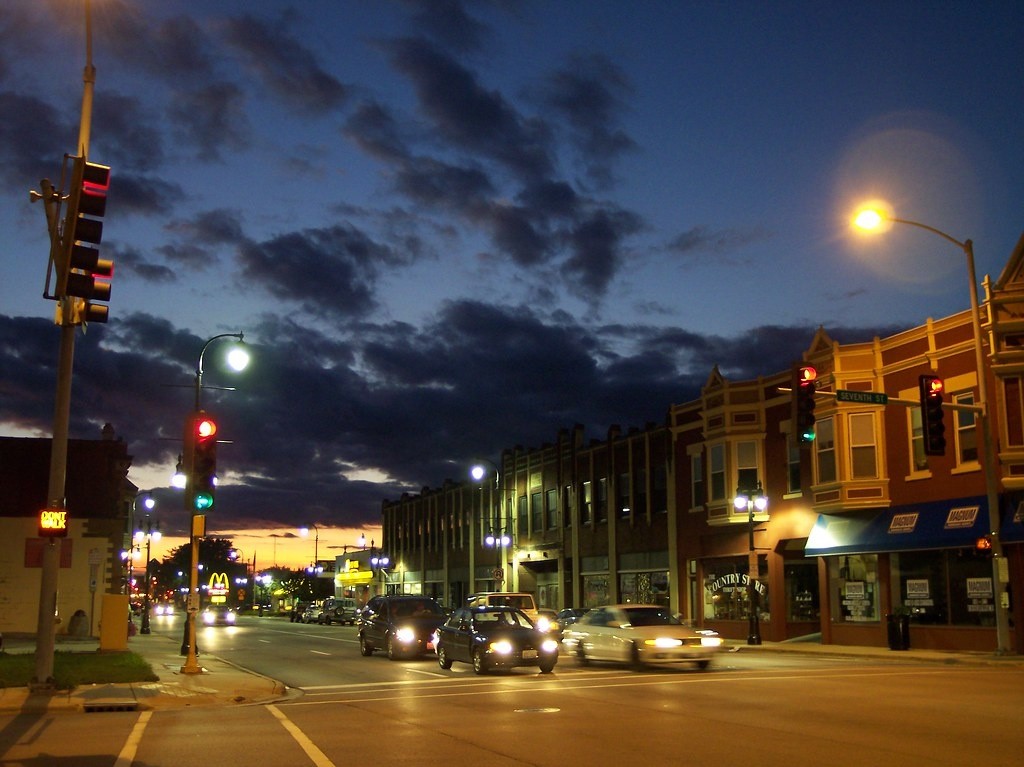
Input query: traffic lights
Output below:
<box><xmin>79</xmin><ymin>256</ymin><xmax>114</xmax><ymax>324</ymax></box>
<box><xmin>790</xmin><ymin>363</ymin><xmax>817</xmax><ymax>443</ymax></box>
<box><xmin>65</xmin><ymin>154</ymin><xmax>113</xmax><ymax>301</ymax></box>
<box><xmin>918</xmin><ymin>372</ymin><xmax>947</xmax><ymax>457</ymax></box>
<box><xmin>975</xmin><ymin>533</ymin><xmax>993</xmax><ymax>552</ymax></box>
<box><xmin>190</xmin><ymin>418</ymin><xmax>218</xmax><ymax>513</ymax></box>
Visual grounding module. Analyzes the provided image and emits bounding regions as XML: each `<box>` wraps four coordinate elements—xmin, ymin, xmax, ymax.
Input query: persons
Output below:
<box><xmin>495</xmin><ymin>615</ymin><xmax>511</xmax><ymax>628</ymax></box>
<box><xmin>413</xmin><ymin>602</ymin><xmax>432</xmax><ymax>616</ymax></box>
<box><xmin>385</xmin><ymin>603</ymin><xmax>400</xmax><ymax>619</ymax></box>
<box><xmin>290</xmin><ymin>607</ymin><xmax>303</xmax><ymax>623</ymax></box>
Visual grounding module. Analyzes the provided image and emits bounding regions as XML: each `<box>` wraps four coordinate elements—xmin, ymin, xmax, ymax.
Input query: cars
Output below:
<box><xmin>200</xmin><ymin>604</ymin><xmax>237</xmax><ymax>627</ymax></box>
<box><xmin>562</xmin><ymin>604</ymin><xmax>724</xmax><ymax>669</ymax></box>
<box><xmin>431</xmin><ymin>604</ymin><xmax>560</xmax><ymax>676</ymax></box>
<box><xmin>289</xmin><ymin>597</ymin><xmax>359</xmax><ymax>625</ymax></box>
<box><xmin>537</xmin><ymin>607</ymin><xmax>610</xmax><ymax>640</ymax></box>
<box><xmin>154</xmin><ymin>604</ymin><xmax>174</xmax><ymax>616</ymax></box>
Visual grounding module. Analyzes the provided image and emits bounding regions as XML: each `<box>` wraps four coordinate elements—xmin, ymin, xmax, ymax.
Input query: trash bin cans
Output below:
<box><xmin>886</xmin><ymin>615</ymin><xmax>911</xmax><ymax>651</ymax></box>
<box><xmin>70</xmin><ymin>610</ymin><xmax>89</xmax><ymax>636</ymax></box>
<box><xmin>101</xmin><ymin>594</ymin><xmax>130</xmax><ymax>650</ymax></box>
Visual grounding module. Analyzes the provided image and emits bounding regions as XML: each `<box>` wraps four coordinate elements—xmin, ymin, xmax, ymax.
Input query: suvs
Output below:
<box><xmin>356</xmin><ymin>593</ymin><xmax>451</xmax><ymax>660</ymax></box>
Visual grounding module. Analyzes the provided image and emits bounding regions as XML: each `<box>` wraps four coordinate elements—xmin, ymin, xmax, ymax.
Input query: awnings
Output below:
<box><xmin>805</xmin><ymin>488</ymin><xmax>1024</xmax><ymax>559</ymax></box>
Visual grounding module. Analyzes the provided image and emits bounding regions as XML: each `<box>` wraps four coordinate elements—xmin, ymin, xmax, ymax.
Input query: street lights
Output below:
<box><xmin>135</xmin><ymin>519</ymin><xmax>162</xmax><ymax>633</ymax></box>
<box><xmin>126</xmin><ymin>490</ymin><xmax>155</xmax><ymax>625</ymax></box>
<box><xmin>849</xmin><ymin>205</ymin><xmax>1015</xmax><ymax>659</ymax></box>
<box><xmin>179</xmin><ymin>330</ymin><xmax>249</xmax><ymax>658</ymax></box>
<box><xmin>470</xmin><ymin>456</ymin><xmax>503</xmax><ymax>594</ymax></box>
<box><xmin>731</xmin><ymin>498</ymin><xmax>766</xmax><ymax>647</ymax></box>
<box><xmin>299</xmin><ymin>520</ymin><xmax>319</xmax><ymax>570</ymax></box>
